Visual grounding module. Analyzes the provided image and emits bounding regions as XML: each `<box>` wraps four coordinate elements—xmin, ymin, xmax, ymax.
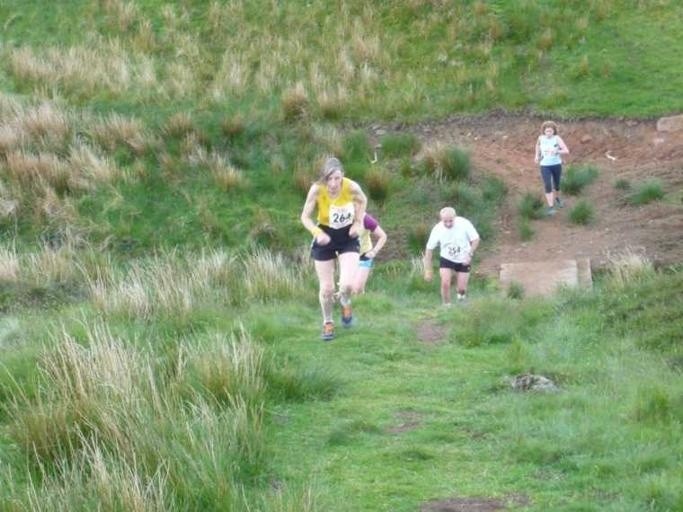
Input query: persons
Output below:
<box><xmin>352</xmin><ymin>213</ymin><xmax>388</xmax><ymax>297</ymax></box>
<box><xmin>301</xmin><ymin>156</ymin><xmax>369</xmax><ymax>341</ymax></box>
<box><xmin>535</xmin><ymin>121</ymin><xmax>569</xmax><ymax>216</ymax></box>
<box><xmin>422</xmin><ymin>206</ymin><xmax>481</xmax><ymax>307</ymax></box>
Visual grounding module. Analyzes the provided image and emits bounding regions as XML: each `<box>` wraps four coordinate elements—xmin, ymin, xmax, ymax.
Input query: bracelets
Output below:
<box><xmin>308</xmin><ymin>226</ymin><xmax>322</xmax><ymax>237</ymax></box>
<box><xmin>468</xmin><ymin>252</ymin><xmax>474</xmax><ymax>257</ymax></box>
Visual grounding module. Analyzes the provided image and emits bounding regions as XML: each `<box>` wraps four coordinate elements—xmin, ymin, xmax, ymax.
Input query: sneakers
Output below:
<box><xmin>340</xmin><ymin>298</ymin><xmax>353</xmax><ymax>324</ymax></box>
<box><xmin>323</xmin><ymin>321</ymin><xmax>335</xmax><ymax>340</ymax></box>
<box><xmin>547</xmin><ymin>206</ymin><xmax>556</xmax><ymax>216</ymax></box>
<box><xmin>556</xmin><ymin>197</ymin><xmax>563</xmax><ymax>208</ymax></box>
